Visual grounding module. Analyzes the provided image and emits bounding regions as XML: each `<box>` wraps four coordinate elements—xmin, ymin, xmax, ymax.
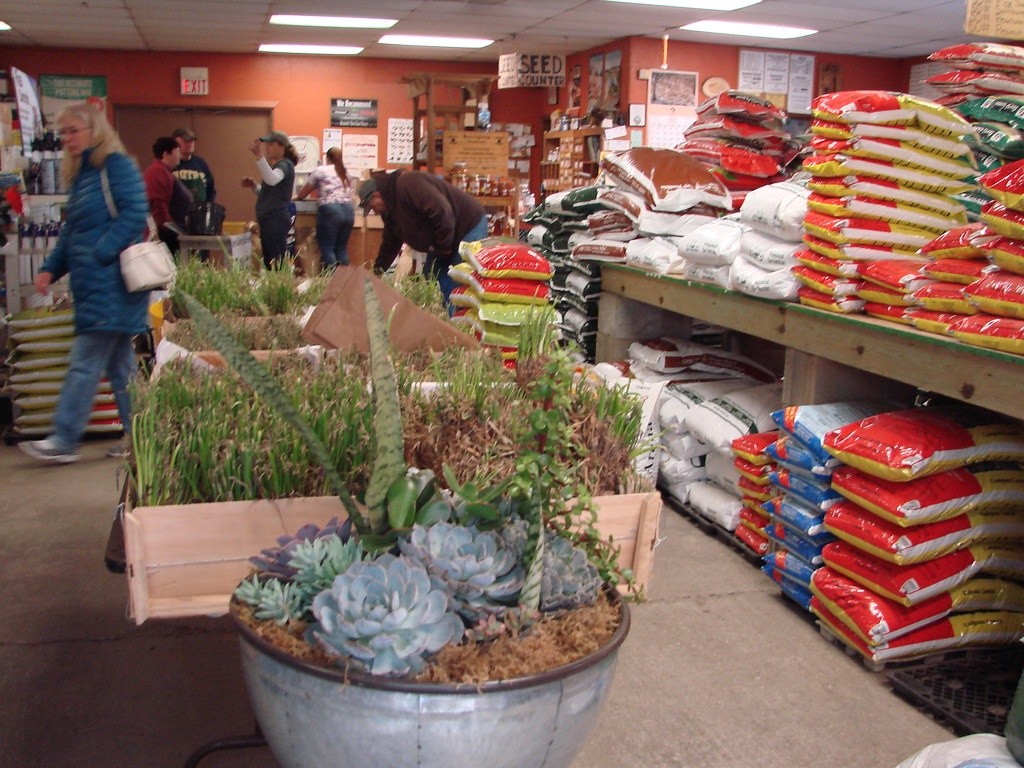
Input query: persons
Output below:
<box><xmin>358</xmin><ymin>171</ymin><xmax>488</xmax><ymax>317</ymax></box>
<box><xmin>242</xmin><ymin>131</ymin><xmax>300</xmax><ymax>270</ymax></box>
<box><xmin>19</xmin><ymin>102</ymin><xmax>149</xmax><ymax>461</ymax></box>
<box><xmin>145</xmin><ymin>136</ymin><xmax>193</xmax><ymax>255</ymax></box>
<box><xmin>291</xmin><ymin>147</ymin><xmax>355</xmax><ymax>274</ymax></box>
<box><xmin>172</xmin><ymin>128</ymin><xmax>216</xmax><ymax>259</ymax></box>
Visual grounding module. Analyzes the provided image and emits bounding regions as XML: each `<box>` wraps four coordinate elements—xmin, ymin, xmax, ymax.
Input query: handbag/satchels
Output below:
<box><xmin>120</xmin><ymin>240</ymin><xmax>177</xmax><ymax>291</ymax></box>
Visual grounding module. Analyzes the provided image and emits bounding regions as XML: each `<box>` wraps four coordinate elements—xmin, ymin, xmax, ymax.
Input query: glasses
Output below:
<box><xmin>56</xmin><ymin>126</ymin><xmax>91</xmax><ymax>138</ymax></box>
<box><xmin>358</xmin><ymin>191</ymin><xmax>374</xmax><ymax>207</ymax></box>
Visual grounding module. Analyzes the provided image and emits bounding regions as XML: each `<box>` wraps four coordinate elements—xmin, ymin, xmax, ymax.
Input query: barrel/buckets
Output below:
<box><xmin>184</xmin><ymin>201</ymin><xmax>224</xmax><ymax>237</ymax></box>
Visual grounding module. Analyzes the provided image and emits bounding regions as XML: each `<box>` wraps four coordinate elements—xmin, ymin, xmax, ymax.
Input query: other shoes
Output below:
<box><xmin>17</xmin><ymin>438</ymin><xmax>82</xmax><ymax>463</ymax></box>
<box><xmin>107</xmin><ymin>442</ymin><xmax>131</xmax><ymax>457</ymax></box>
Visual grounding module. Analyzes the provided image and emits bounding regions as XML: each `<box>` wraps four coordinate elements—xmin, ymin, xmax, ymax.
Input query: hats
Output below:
<box><xmin>259</xmin><ymin>131</ymin><xmax>289</xmax><ymax>146</ymax></box>
<box><xmin>172</xmin><ymin>127</ymin><xmax>197</xmax><ymax>141</ymax></box>
<box><xmin>357</xmin><ymin>179</ymin><xmax>380</xmax><ymax>217</ymax></box>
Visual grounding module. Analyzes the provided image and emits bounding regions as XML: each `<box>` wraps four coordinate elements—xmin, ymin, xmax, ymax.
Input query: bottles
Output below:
<box><xmin>448</xmin><ymin>162</ymin><xmax>514</xmax><ymax>197</ymax></box>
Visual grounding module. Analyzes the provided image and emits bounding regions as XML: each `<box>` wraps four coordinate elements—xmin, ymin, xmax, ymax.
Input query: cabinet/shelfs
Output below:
<box><xmin>402</xmin><ymin>67</ymin><xmax>519</xmax><ymax>239</ymax></box>
<box><xmin>0</xmin><ymin>147</ymin><xmax>75</xmax><ymax>315</ymax></box>
<box><xmin>540</xmin><ymin>126</ymin><xmax>605</xmax><ymax>203</ymax></box>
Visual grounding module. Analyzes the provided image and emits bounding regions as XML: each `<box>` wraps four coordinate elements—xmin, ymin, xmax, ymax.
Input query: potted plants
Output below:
<box><xmin>118</xmin><ymin>247</ymin><xmax>660</xmax><ymax>768</ymax></box>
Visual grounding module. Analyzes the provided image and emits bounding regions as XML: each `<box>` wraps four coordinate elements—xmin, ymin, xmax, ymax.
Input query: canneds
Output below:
<box><xmin>450</xmin><ymin>162</ymin><xmax>515</xmax><ymax>239</ymax></box>
